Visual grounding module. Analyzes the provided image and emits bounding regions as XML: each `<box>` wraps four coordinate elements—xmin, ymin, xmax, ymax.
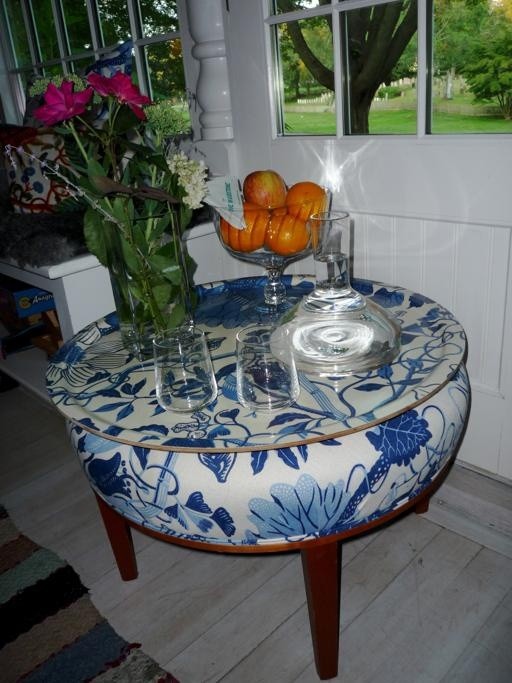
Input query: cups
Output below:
<box><xmin>149</xmin><ymin>324</ymin><xmax>219</xmax><ymax>413</ymax></box>
<box><xmin>234</xmin><ymin>321</ymin><xmax>301</xmax><ymax>413</ymax></box>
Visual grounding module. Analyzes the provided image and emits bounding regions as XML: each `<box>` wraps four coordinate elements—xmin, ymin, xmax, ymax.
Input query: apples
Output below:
<box><xmin>243</xmin><ymin>170</ymin><xmax>287</xmax><ymax>209</ymax></box>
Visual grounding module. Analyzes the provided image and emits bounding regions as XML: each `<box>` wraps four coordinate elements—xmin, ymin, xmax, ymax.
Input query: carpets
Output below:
<box><xmin>0</xmin><ymin>503</ymin><xmax>177</xmax><ymax>681</ymax></box>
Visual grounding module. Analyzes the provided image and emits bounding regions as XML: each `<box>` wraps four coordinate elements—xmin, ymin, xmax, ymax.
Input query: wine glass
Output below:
<box><xmin>210</xmin><ymin>189</ymin><xmax>335</xmax><ymax>329</ymax></box>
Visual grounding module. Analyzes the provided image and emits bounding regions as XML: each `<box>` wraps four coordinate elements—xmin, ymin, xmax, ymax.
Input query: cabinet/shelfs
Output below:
<box><xmin>1</xmin><ymin>220</ymin><xmax>264</xmax><ymax>412</ymax></box>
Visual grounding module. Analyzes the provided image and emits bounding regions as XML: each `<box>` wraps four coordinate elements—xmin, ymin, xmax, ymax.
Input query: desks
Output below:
<box><xmin>45</xmin><ymin>272</ymin><xmax>472</xmax><ymax>680</ymax></box>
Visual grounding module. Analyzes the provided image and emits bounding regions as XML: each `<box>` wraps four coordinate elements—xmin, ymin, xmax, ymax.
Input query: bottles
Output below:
<box><xmin>265</xmin><ymin>210</ymin><xmax>403</xmax><ymax>373</ymax></box>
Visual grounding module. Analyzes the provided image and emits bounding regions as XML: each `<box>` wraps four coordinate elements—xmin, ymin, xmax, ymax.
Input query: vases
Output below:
<box><xmin>100</xmin><ymin>209</ymin><xmax>194</xmax><ymax>362</ymax></box>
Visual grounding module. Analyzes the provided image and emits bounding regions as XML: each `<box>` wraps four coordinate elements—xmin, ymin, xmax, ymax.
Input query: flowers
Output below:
<box><xmin>3</xmin><ymin>65</ymin><xmax>211</xmax><ymax>346</ymax></box>
